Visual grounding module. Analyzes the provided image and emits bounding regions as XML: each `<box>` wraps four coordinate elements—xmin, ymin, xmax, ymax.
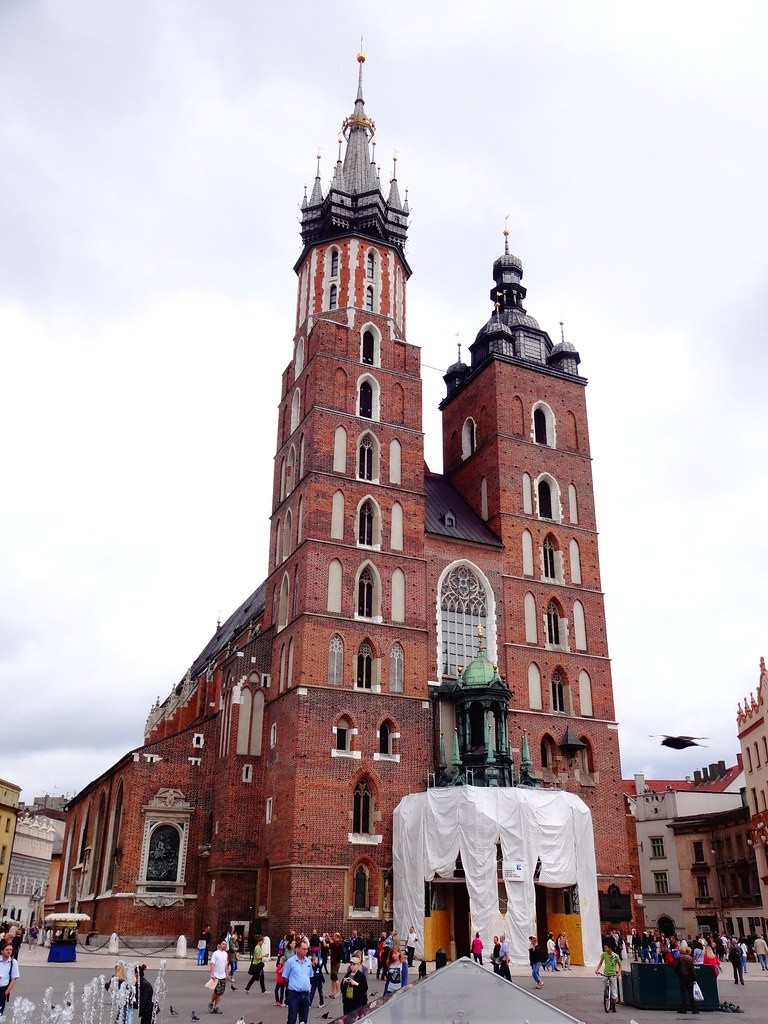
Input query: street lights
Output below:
<box><xmin>710</xmin><ymin>846</ymin><xmax>726</xmax><ymax>935</ymax></box>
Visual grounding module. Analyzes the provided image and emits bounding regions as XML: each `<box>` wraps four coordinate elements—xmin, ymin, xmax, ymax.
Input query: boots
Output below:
<box><xmin>611</xmin><ymin>999</ymin><xmax>617</xmax><ymax>1012</ymax></box>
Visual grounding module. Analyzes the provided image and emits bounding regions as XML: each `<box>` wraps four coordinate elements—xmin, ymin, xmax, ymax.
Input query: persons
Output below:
<box><xmin>542</xmin><ymin>931</ymin><xmax>572</xmax><ymax>972</ymax></box>
<box><xmin>379</xmin><ymin>945</ymin><xmax>409</xmax><ymax>1001</ymax></box>
<box><xmin>498</xmin><ymin>934</ymin><xmax>513</xmax><ymax>983</ymax></box>
<box><xmin>471</xmin><ymin>932</ymin><xmax>484</xmax><ymax>966</ymax></box>
<box><xmin>283</xmin><ymin>939</ymin><xmax>315</xmax><ymax>1024</ymax></box>
<box><xmin>206</xmin><ymin>939</ymin><xmax>231</xmax><ymax>1014</ymax></box>
<box><xmin>529</xmin><ymin>936</ymin><xmax>544</xmax><ymax>989</ymax></box>
<box><xmin>674</xmin><ymin>945</ymin><xmax>699</xmax><ymax>1014</ymax></box>
<box><xmin>197</xmin><ymin>924</ymin><xmax>398</xmax><ymax>1008</ymax></box>
<box><xmin>600</xmin><ymin>926</ymin><xmax>768</xmax><ymax>986</ymax></box>
<box><xmin>404</xmin><ymin>926</ymin><xmax>418</xmax><ymax>967</ymax></box>
<box><xmin>0</xmin><ymin>919</ymin><xmax>26</xmax><ymax>962</ymax></box>
<box><xmin>28</xmin><ymin>922</ymin><xmax>39</xmax><ymax>951</ymax></box>
<box><xmin>340</xmin><ymin>957</ymin><xmax>368</xmax><ymax>1017</ymax></box>
<box><xmin>595</xmin><ymin>942</ymin><xmax>622</xmax><ymax>1013</ymax></box>
<box><xmin>104</xmin><ymin>960</ymin><xmax>133</xmax><ymax>1024</ymax></box>
<box><xmin>0</xmin><ymin>942</ymin><xmax>20</xmax><ymax>1018</ymax></box>
<box><xmin>130</xmin><ymin>964</ymin><xmax>157</xmax><ymax>1024</ymax></box>
<box><xmin>492</xmin><ymin>936</ymin><xmax>501</xmax><ymax>974</ymax></box>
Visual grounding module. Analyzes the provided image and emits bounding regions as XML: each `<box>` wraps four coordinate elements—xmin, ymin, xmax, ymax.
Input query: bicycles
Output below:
<box><xmin>596</xmin><ymin>973</ymin><xmax>620</xmax><ymax>1013</ymax></box>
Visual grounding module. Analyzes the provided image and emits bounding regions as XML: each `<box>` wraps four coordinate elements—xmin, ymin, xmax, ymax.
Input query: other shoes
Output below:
<box><xmin>546</xmin><ymin>965</ymin><xmax>549</xmax><ymax>971</ymax></box>
<box><xmin>273</xmin><ymin>1001</ymin><xmax>283</xmax><ymax>1006</ymax></box>
<box><xmin>325</xmin><ymin>993</ymin><xmax>342</xmax><ymax>999</ymax></box>
<box><xmin>370</xmin><ymin>972</ymin><xmax>372</xmax><ymax>974</ymax></box>
<box><xmin>553</xmin><ymin>967</ymin><xmax>560</xmax><ymax>972</ymax></box>
<box><xmin>244</xmin><ymin>989</ymin><xmax>250</xmax><ymax>994</ymax></box>
<box><xmin>634</xmin><ymin>959</ymin><xmax>638</xmax><ymax>961</ymax></box>
<box><xmin>376</xmin><ymin>978</ymin><xmax>380</xmax><ymax>980</ymax></box>
<box><xmin>533</xmin><ymin>982</ymin><xmax>544</xmax><ymax>990</ymax></box>
<box><xmin>319</xmin><ymin>1005</ymin><xmax>325</xmax><ymax>1008</ymax></box>
<box><xmin>229</xmin><ymin>976</ymin><xmax>235</xmax><ymax>982</ymax></box>
<box><xmin>381</xmin><ymin>979</ymin><xmax>387</xmax><ymax>981</ymax></box>
<box><xmin>261</xmin><ymin>991</ymin><xmax>271</xmax><ymax>994</ymax></box>
<box><xmin>541</xmin><ymin>964</ymin><xmax>546</xmax><ymax>971</ymax></box>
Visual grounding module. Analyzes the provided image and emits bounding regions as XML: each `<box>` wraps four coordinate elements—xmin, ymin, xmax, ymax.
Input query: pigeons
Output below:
<box><xmin>190</xmin><ymin>1010</ymin><xmax>200</xmax><ymax>1022</ymax></box>
<box><xmin>321</xmin><ymin>1011</ymin><xmax>330</xmax><ymax>1020</ymax></box>
<box><xmin>169</xmin><ymin>1005</ymin><xmax>179</xmax><ymax>1016</ymax></box>
<box><xmin>230</xmin><ymin>984</ymin><xmax>237</xmax><ymax>992</ymax></box>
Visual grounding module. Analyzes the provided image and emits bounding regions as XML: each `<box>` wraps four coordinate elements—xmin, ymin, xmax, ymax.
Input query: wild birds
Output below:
<box><xmin>651</xmin><ymin>731</ymin><xmax>707</xmax><ymax>751</ymax></box>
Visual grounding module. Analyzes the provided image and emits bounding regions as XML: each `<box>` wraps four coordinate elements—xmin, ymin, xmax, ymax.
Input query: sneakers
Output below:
<box><xmin>213</xmin><ymin>1007</ymin><xmax>222</xmax><ymax>1014</ymax></box>
<box><xmin>207</xmin><ymin>1001</ymin><xmax>214</xmax><ymax>1013</ymax></box>
<box><xmin>281</xmin><ymin>1003</ymin><xmax>288</xmax><ymax>1007</ymax></box>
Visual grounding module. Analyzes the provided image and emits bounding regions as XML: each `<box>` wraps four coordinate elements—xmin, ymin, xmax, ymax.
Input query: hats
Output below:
<box><xmin>350</xmin><ymin>957</ymin><xmax>360</xmax><ymax>963</ymax></box>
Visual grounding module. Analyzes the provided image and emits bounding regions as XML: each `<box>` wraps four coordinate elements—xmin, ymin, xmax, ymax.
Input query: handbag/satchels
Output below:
<box><xmin>346</xmin><ymin>986</ymin><xmax>353</xmax><ymax>999</ymax></box>
<box><xmin>205</xmin><ymin>979</ymin><xmax>219</xmax><ymax>990</ymax></box>
<box><xmin>553</xmin><ymin>948</ymin><xmax>558</xmax><ymax>956</ymax></box>
<box><xmin>715</xmin><ymin>963</ymin><xmax>725</xmax><ymax>975</ymax></box>
<box><xmin>373</xmin><ymin>948</ymin><xmax>381</xmax><ymax>958</ymax></box>
<box><xmin>728</xmin><ymin>944</ymin><xmax>739</xmax><ymax>963</ymax></box>
<box><xmin>692</xmin><ymin>981</ymin><xmax>704</xmax><ymax>1001</ymax></box>
<box><xmin>248</xmin><ymin>958</ymin><xmax>265</xmax><ymax>977</ymax></box>
